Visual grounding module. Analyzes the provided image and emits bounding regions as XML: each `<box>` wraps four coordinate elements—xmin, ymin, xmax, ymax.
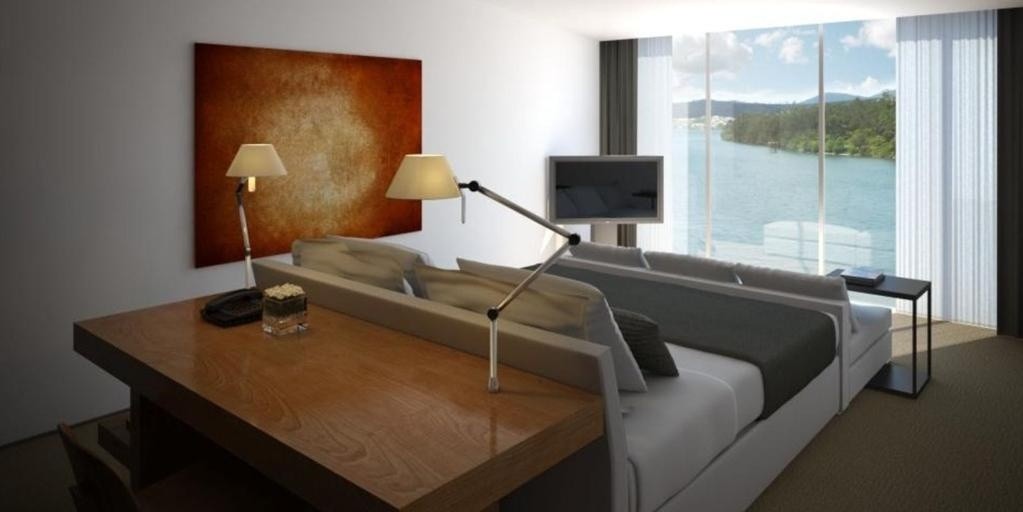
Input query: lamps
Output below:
<box><xmin>385</xmin><ymin>154</ymin><xmax>582</xmax><ymax>394</ymax></box>
<box><xmin>225</xmin><ymin>144</ymin><xmax>288</xmax><ymax>288</ymax></box>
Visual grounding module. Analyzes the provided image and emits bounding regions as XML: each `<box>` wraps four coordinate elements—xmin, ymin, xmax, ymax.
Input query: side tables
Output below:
<box><xmin>825</xmin><ymin>268</ymin><xmax>931</xmax><ymax>399</ymax></box>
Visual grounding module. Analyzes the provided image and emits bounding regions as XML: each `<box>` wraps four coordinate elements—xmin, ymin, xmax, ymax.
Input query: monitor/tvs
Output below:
<box><xmin>547</xmin><ymin>154</ymin><xmax>664</xmax><ymax>224</ymax></box>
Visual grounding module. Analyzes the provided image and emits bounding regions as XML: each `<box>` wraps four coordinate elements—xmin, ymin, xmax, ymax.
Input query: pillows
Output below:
<box><xmin>644</xmin><ymin>252</ymin><xmax>740</xmax><ymax>284</ymax></box>
<box><xmin>734</xmin><ymin>263</ymin><xmax>861</xmax><ymax>334</ymax></box>
<box><xmin>569</xmin><ymin>240</ymin><xmax>651</xmax><ymax>270</ymax></box>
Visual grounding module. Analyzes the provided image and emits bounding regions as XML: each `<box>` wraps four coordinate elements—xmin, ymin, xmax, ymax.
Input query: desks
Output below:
<box><xmin>74</xmin><ymin>287</ymin><xmax>605</xmax><ymax>512</ymax></box>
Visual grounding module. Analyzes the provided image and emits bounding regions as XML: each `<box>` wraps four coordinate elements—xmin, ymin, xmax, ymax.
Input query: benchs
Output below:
<box><xmin>554</xmin><ymin>257</ymin><xmax>892</xmax><ymax>413</ymax></box>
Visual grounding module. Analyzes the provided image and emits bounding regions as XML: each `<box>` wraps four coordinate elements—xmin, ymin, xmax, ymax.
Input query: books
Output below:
<box><xmin>841</xmin><ymin>263</ymin><xmax>886</xmax><ymax>287</ymax></box>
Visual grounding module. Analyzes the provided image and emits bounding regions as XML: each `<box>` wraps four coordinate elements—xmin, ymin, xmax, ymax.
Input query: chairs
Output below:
<box><xmin>58</xmin><ymin>424</ymin><xmax>320</xmax><ymax>512</ymax></box>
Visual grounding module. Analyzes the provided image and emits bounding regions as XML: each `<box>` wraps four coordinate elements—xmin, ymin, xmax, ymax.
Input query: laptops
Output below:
<box><xmin>839</xmin><ymin>266</ymin><xmax>883</xmax><ymax>286</ymax></box>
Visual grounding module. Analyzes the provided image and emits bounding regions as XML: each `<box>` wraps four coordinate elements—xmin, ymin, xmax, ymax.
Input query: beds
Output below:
<box><xmin>250</xmin><ymin>262</ymin><xmax>841</xmax><ymax>512</ymax></box>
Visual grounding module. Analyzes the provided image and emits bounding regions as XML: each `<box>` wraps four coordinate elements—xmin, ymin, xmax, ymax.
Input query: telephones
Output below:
<box><xmin>200</xmin><ymin>287</ymin><xmax>264</xmax><ymax>327</ymax></box>
